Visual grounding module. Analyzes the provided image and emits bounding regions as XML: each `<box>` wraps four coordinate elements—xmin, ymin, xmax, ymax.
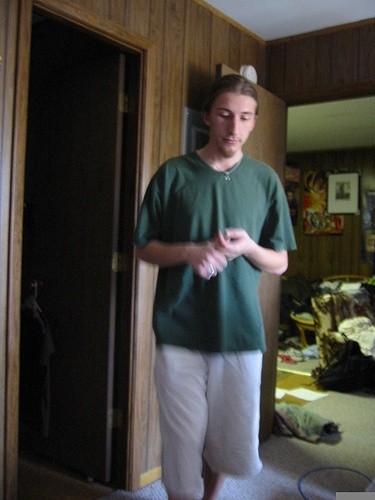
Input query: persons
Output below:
<box><xmin>132</xmin><ymin>77</ymin><xmax>298</xmax><ymax>500</ymax></box>
<box><xmin>336</xmin><ymin>182</ymin><xmax>349</xmax><ymax>200</ymax></box>
<box><xmin>285</xmin><ymin>189</ymin><xmax>297</xmax><ymax>225</ymax></box>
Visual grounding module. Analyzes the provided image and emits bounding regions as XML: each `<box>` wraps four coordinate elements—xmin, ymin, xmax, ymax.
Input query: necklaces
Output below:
<box><xmin>202</xmin><ymin>143</ymin><xmax>243</xmax><ymax>181</ymax></box>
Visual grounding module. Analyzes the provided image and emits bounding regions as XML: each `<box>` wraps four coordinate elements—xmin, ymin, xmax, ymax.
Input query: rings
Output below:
<box><xmin>208</xmin><ymin>267</ymin><xmax>216</xmax><ymax>279</ymax></box>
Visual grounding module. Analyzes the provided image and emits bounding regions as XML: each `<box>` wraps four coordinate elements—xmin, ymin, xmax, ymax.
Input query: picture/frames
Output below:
<box><xmin>325</xmin><ymin>169</ymin><xmax>362</xmax><ymax>216</ymax></box>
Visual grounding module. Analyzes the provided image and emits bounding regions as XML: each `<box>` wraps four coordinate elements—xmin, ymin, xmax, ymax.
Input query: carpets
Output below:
<box><xmin>98</xmin><ymin>387</ymin><xmax>375</xmax><ymax>500</ymax></box>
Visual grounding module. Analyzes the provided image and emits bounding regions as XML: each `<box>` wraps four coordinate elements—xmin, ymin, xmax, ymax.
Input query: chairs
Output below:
<box><xmin>288</xmin><ymin>274</ymin><xmax>375</xmax><ymax>353</ymax></box>
